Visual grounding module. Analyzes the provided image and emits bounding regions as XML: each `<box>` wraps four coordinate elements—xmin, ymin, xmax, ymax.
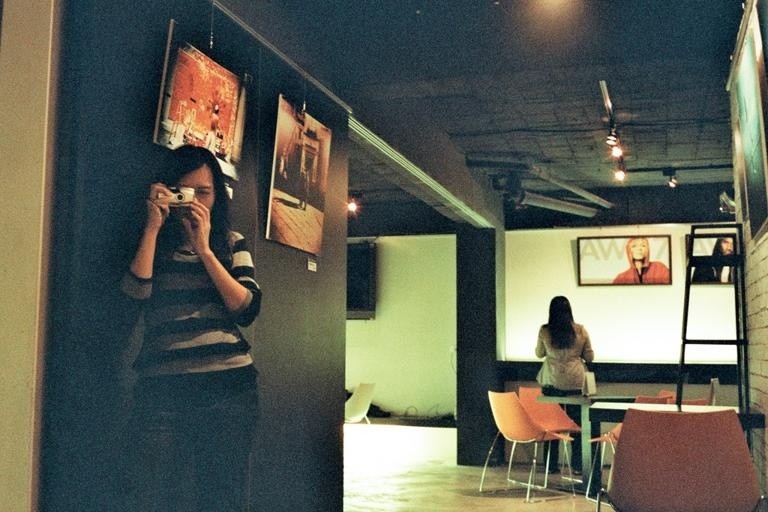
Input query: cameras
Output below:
<box><xmin>158</xmin><ymin>186</ymin><xmax>194</xmax><ymax>207</ymax></box>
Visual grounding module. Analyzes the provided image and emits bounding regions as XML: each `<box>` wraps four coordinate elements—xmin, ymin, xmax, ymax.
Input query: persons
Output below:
<box><xmin>693</xmin><ymin>237</ymin><xmax>737</xmax><ymax>282</ymax></box>
<box><xmin>535</xmin><ymin>297</ymin><xmax>595</xmax><ymax>476</ymax></box>
<box><xmin>109</xmin><ymin>144</ymin><xmax>263</xmax><ymax>511</ymax></box>
<box><xmin>612</xmin><ymin>237</ymin><xmax>670</xmax><ymax>285</ymax></box>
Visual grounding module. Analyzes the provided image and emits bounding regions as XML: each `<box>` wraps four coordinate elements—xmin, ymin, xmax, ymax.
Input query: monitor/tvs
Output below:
<box><xmin>347</xmin><ymin>242</ymin><xmax>376</xmax><ymax>320</ymax></box>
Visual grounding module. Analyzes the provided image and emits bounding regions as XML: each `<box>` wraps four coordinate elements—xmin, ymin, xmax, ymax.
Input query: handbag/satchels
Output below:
<box><xmin>581</xmin><ymin>372</ymin><xmax>597</xmax><ymax>396</ymax></box>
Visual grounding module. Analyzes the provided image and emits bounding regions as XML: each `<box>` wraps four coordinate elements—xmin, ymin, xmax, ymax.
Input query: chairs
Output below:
<box><xmin>478</xmin><ymin>387</ymin><xmax>759</xmax><ymax>512</ymax></box>
<box><xmin>344</xmin><ymin>383</ymin><xmax>375</xmax><ymax>426</ymax></box>
